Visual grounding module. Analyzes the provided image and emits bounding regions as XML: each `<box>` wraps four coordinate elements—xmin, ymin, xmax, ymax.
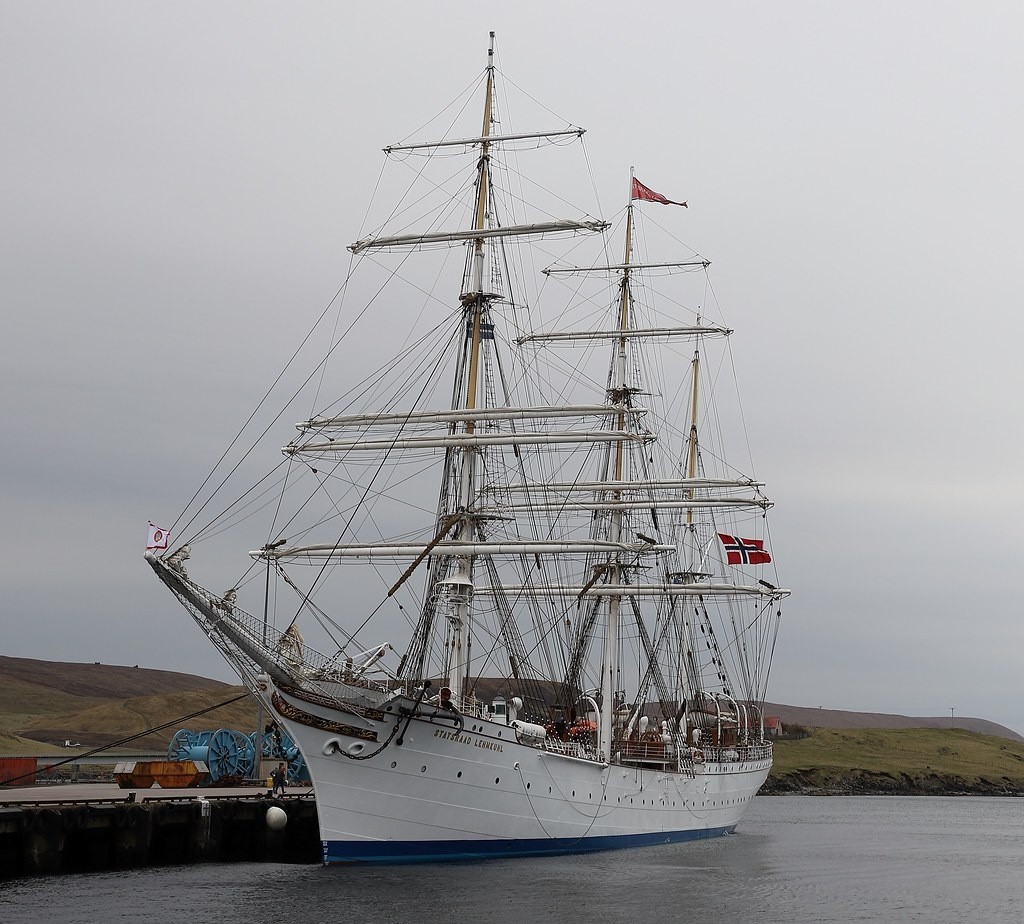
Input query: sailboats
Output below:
<box><xmin>137</xmin><ymin>28</ymin><xmax>798</xmax><ymax>870</ymax></box>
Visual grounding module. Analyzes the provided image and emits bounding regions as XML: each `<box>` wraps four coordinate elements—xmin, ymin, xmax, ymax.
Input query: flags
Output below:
<box><xmin>146</xmin><ymin>523</ymin><xmax>170</xmax><ymax>549</ymax></box>
<box><xmin>631</xmin><ymin>176</ymin><xmax>688</xmax><ymax>208</ymax></box>
<box><xmin>717</xmin><ymin>533</ymin><xmax>772</xmax><ymax>565</ymax></box>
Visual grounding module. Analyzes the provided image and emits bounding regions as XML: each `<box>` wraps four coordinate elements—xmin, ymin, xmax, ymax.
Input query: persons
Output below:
<box><xmin>571</xmin><ymin>704</ymin><xmax>578</xmax><ymax>728</ymax></box>
<box><xmin>270</xmin><ymin>765</ymin><xmax>287</xmax><ymax>793</ymax></box>
